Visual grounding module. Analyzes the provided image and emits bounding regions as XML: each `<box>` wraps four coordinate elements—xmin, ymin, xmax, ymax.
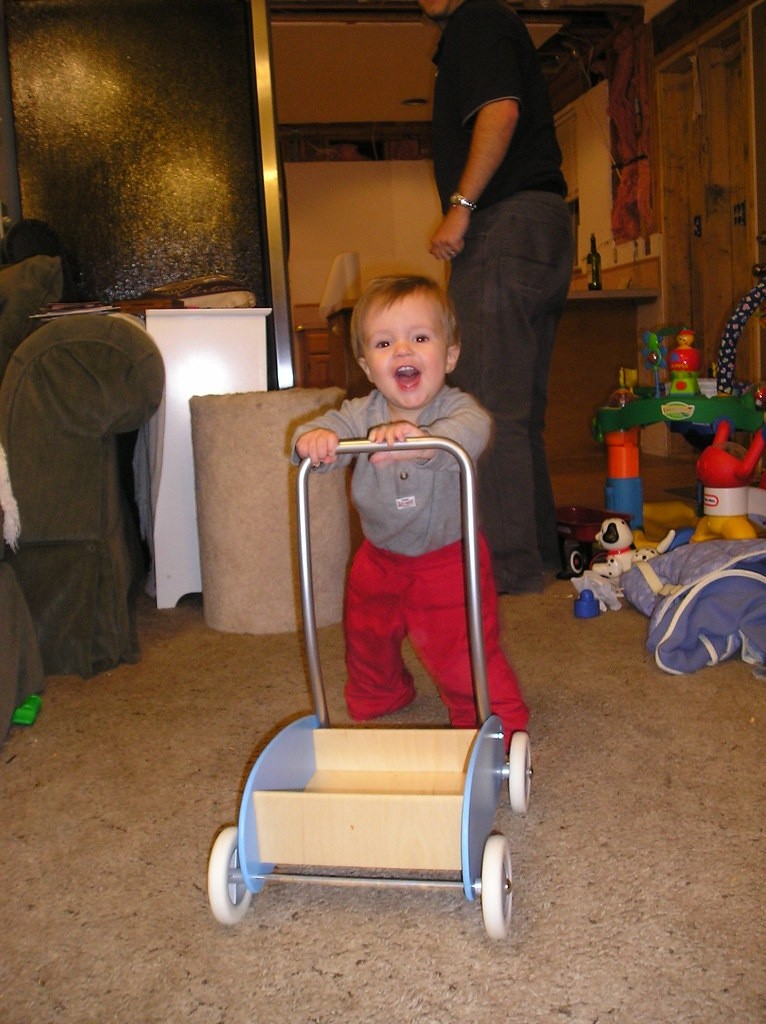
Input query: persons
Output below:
<box><xmin>288</xmin><ymin>276</ymin><xmax>530</xmax><ymax>758</ymax></box>
<box><xmin>414</xmin><ymin>0</ymin><xmax>565</xmax><ymax>594</ymax></box>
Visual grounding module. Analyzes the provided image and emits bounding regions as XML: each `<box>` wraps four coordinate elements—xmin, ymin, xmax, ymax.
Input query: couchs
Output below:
<box><xmin>0</xmin><ymin>254</ymin><xmax>165</xmax><ymax>678</ymax></box>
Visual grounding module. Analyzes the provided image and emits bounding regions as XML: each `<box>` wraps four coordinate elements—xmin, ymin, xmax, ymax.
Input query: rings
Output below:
<box><xmin>449</xmin><ymin>250</ymin><xmax>455</xmax><ymax>256</ymax></box>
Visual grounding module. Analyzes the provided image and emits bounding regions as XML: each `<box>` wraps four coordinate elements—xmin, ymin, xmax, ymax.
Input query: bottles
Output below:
<box><xmin>587</xmin><ymin>234</ymin><xmax>602</xmax><ymax>290</ymax></box>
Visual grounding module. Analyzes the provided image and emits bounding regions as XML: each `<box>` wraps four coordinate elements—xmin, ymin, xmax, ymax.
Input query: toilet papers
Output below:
<box><xmin>317</xmin><ymin>250</ymin><xmax>361</xmax><ymax>320</ymax></box>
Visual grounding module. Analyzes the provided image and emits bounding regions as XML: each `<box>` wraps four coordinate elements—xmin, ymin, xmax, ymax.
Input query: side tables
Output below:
<box><xmin>141</xmin><ymin>309</ymin><xmax>273</xmax><ymax>612</ymax></box>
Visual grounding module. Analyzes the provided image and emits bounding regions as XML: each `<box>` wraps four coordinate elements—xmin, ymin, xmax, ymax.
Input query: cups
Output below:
<box><xmin>604</xmin><ymin>477</ymin><xmax>644</xmax><ymax>531</ymax></box>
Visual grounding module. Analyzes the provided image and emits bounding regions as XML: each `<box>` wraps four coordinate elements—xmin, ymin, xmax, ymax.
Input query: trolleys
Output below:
<box><xmin>205</xmin><ymin>436</ymin><xmax>532</xmax><ymax>941</ymax></box>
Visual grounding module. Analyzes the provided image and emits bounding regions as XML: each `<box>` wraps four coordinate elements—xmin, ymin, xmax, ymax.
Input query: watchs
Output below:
<box><xmin>449</xmin><ymin>191</ymin><xmax>478</xmax><ymax>211</ymax></box>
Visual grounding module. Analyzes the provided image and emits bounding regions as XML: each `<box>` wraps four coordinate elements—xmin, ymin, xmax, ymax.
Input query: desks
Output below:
<box><xmin>541</xmin><ymin>290</ymin><xmax>657</xmax><ymax>459</ymax></box>
